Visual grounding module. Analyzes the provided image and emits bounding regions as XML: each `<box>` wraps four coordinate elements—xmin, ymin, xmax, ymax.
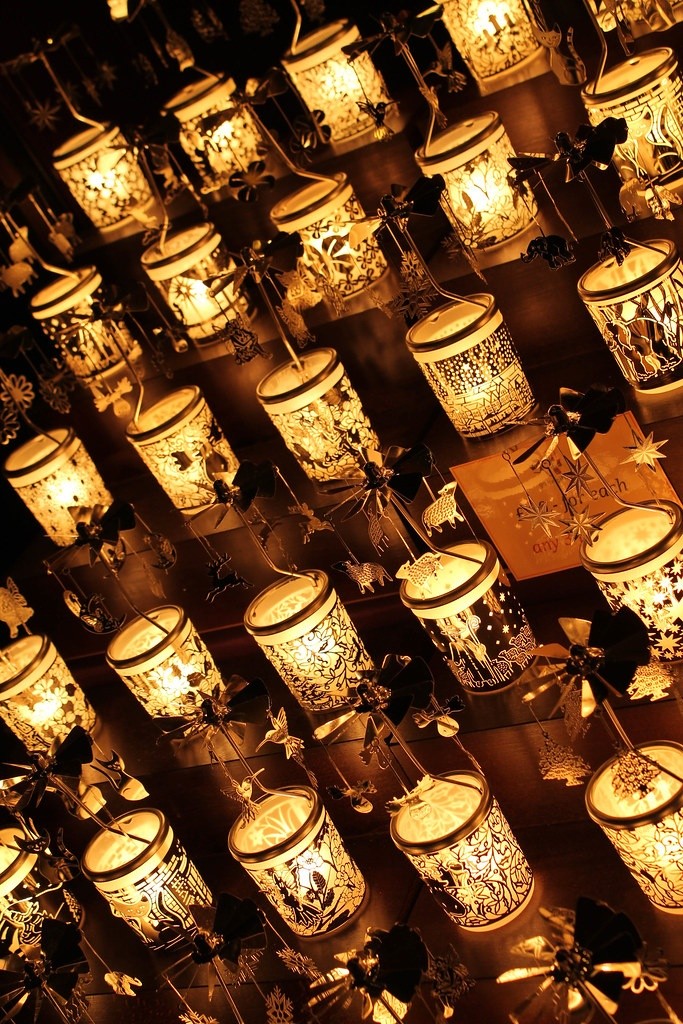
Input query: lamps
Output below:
<box><xmin>0</xmin><ymin>0</ymin><xmax>682</xmax><ymax>1023</ymax></box>
